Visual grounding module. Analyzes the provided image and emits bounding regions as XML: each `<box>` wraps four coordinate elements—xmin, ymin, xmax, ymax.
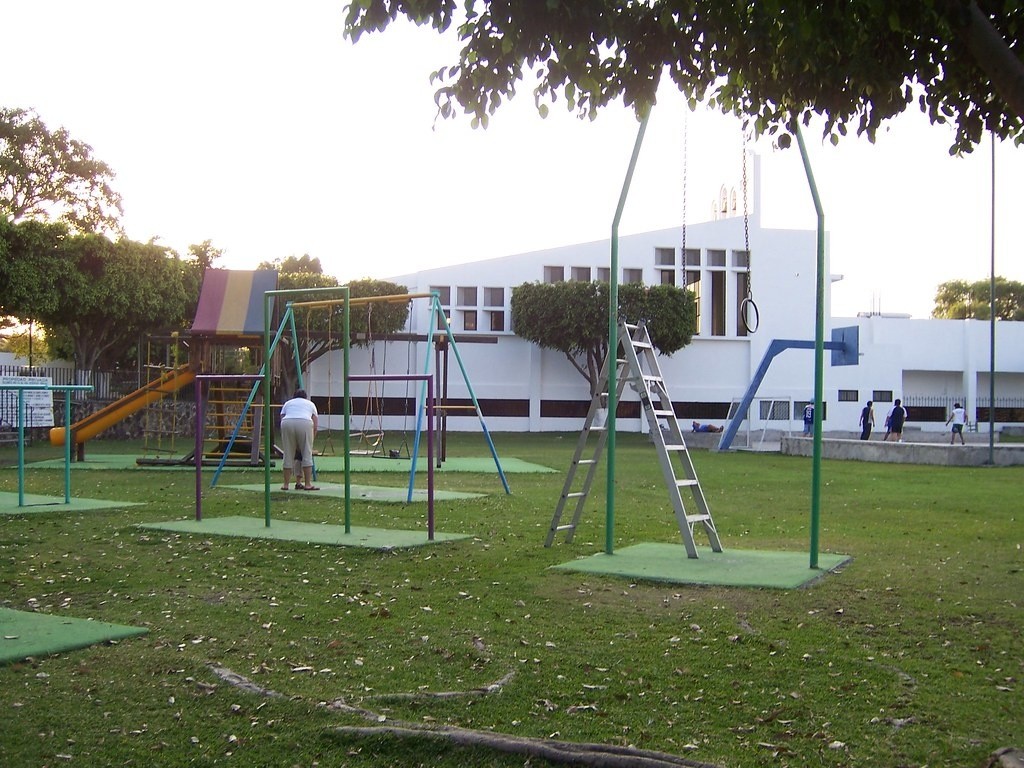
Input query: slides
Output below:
<box><xmin>50</xmin><ymin>362</ymin><xmax>196</xmax><ymax>444</ymax></box>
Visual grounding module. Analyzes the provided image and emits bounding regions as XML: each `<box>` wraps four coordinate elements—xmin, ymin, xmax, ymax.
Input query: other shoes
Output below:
<box><xmin>295</xmin><ymin>484</ymin><xmax>304</xmax><ymax>489</ymax></box>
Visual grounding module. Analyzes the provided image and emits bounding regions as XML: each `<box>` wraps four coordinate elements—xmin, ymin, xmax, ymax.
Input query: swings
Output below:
<box><xmin>305</xmin><ymin>309</ymin><xmax>335</xmax><ymax>457</ymax></box>
<box><xmin>345</xmin><ymin>307</ymin><xmax>390</xmax><ymax>455</ymax></box>
<box><xmin>372</xmin><ymin>302</ymin><xmax>410</xmax><ymax>459</ymax></box>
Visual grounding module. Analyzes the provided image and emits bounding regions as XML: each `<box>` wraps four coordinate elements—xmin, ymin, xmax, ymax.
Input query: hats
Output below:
<box><xmin>810</xmin><ymin>399</ymin><xmax>814</xmax><ymax>403</ymax></box>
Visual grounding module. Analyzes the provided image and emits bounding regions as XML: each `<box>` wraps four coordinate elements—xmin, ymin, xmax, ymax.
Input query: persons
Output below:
<box><xmin>961</xmin><ymin>407</ymin><xmax>968</xmax><ymax>432</ymax></box>
<box><xmin>883</xmin><ymin>416</ymin><xmax>892</xmax><ymax>440</ymax></box>
<box><xmin>802</xmin><ymin>398</ymin><xmax>815</xmax><ymax>437</ymax></box>
<box><xmin>946</xmin><ymin>403</ymin><xmax>965</xmax><ymax>445</ymax></box>
<box><xmin>887</xmin><ymin>399</ymin><xmax>908</xmax><ymax>442</ymax></box>
<box><xmin>859</xmin><ymin>400</ymin><xmax>876</xmax><ymax>440</ymax></box>
<box><xmin>279</xmin><ymin>389</ymin><xmax>320</xmax><ymax>491</ymax></box>
<box><xmin>692</xmin><ymin>421</ymin><xmax>720</xmax><ymax>433</ymax></box>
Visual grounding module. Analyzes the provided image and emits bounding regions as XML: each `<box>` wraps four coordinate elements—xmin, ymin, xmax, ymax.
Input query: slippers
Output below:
<box><xmin>304</xmin><ymin>486</ymin><xmax>320</xmax><ymax>490</ymax></box>
<box><xmin>281</xmin><ymin>486</ymin><xmax>288</xmax><ymax>490</ymax></box>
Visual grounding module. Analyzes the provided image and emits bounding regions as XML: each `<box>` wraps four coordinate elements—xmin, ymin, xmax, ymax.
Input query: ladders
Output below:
<box><xmin>543</xmin><ymin>313</ymin><xmax>724</xmax><ymax>560</ymax></box>
